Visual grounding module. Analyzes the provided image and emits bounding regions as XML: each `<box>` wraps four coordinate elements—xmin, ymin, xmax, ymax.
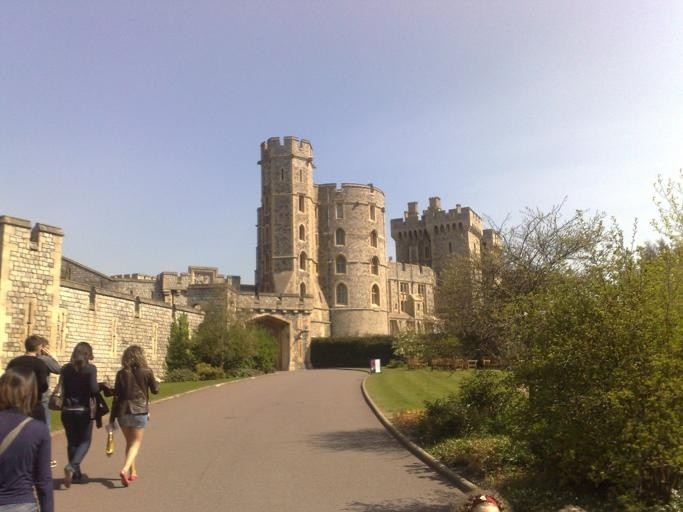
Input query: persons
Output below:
<box><xmin>459</xmin><ymin>490</ymin><xmax>508</xmax><ymax>512</ymax></box>
<box><xmin>0</xmin><ymin>335</ymin><xmax>159</xmax><ymax>512</ymax></box>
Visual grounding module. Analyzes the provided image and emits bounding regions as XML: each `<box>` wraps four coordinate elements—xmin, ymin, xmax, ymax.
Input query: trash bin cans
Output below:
<box><xmin>371</xmin><ymin>358</ymin><xmax>380</xmax><ymax>373</ymax></box>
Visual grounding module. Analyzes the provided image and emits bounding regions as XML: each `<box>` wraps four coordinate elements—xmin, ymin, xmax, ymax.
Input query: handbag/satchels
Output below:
<box><xmin>104</xmin><ymin>432</ymin><xmax>114</xmax><ymax>456</ymax></box>
<box><xmin>48</xmin><ymin>394</ymin><xmax>63</xmax><ymax>410</ymax></box>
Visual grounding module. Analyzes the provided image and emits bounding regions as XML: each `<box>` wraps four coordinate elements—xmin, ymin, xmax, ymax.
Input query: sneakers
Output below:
<box><xmin>64</xmin><ymin>464</ymin><xmax>81</xmax><ymax>489</ymax></box>
<box><xmin>50</xmin><ymin>459</ymin><xmax>57</xmax><ymax>467</ymax></box>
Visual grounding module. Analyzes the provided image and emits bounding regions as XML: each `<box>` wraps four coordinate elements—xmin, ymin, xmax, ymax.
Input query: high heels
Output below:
<box><xmin>119</xmin><ymin>469</ymin><xmax>138</xmax><ymax>486</ymax></box>
